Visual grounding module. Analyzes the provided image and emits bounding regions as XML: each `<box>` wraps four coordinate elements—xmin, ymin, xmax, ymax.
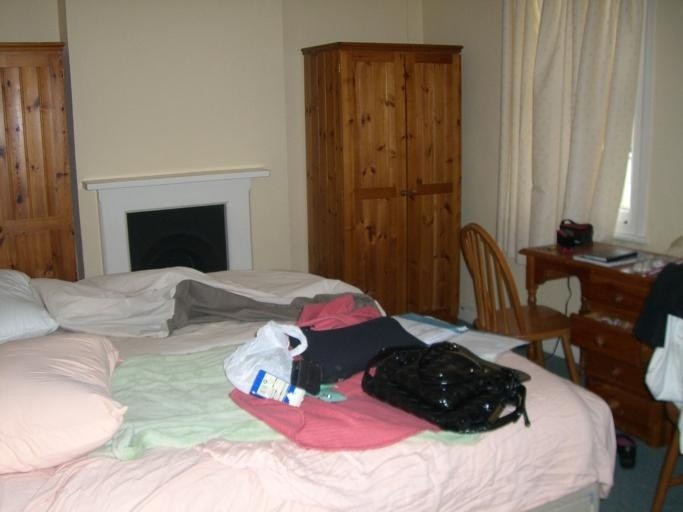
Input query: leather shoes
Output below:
<box><xmin>616</xmin><ymin>428</ymin><xmax>638</xmax><ymax>469</ymax></box>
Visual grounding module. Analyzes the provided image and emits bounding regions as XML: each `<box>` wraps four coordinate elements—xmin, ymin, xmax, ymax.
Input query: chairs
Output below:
<box><xmin>459</xmin><ymin>222</ymin><xmax>579</xmax><ymax>388</ymax></box>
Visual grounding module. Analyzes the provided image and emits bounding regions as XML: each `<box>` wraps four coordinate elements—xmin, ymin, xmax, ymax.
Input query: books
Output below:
<box><xmin>573</xmin><ymin>248</ymin><xmax>640</xmax><ymax>268</ymax></box>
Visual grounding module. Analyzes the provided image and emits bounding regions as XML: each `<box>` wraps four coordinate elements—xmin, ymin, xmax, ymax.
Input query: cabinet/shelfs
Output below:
<box><xmin>299</xmin><ymin>39</ymin><xmax>464</xmax><ymax>321</ymax></box>
<box><xmin>571</xmin><ymin>280</ymin><xmax>667</xmax><ymax>450</ymax></box>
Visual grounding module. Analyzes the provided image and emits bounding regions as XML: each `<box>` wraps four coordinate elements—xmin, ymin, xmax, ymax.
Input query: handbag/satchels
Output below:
<box><xmin>362</xmin><ymin>338</ymin><xmax>530</xmax><ymax>435</ymax></box>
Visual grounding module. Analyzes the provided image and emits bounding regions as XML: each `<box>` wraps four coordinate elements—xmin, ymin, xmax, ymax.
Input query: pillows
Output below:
<box><xmin>0</xmin><ymin>334</ymin><xmax>127</xmax><ymax>476</ymax></box>
<box><xmin>1</xmin><ymin>267</ymin><xmax>60</xmax><ymax>340</ymax></box>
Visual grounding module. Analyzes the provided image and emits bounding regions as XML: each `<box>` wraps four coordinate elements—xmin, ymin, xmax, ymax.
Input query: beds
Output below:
<box><xmin>0</xmin><ymin>266</ymin><xmax>616</xmax><ymax>511</ymax></box>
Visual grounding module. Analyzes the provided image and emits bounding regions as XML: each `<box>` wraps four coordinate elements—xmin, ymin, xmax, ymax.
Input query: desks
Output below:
<box><xmin>521</xmin><ymin>232</ymin><xmax>676</xmax><ymax>289</ymax></box>
